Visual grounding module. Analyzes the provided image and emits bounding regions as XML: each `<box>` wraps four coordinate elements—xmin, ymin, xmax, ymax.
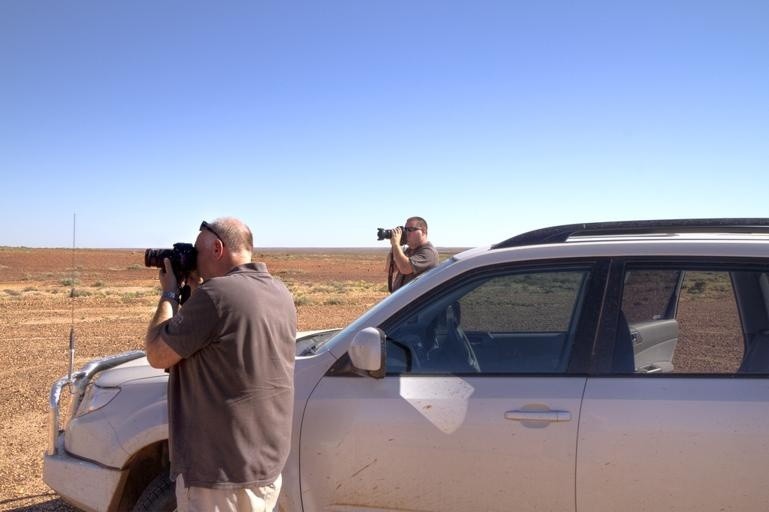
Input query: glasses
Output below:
<box><xmin>405</xmin><ymin>227</ymin><xmax>422</xmax><ymax>232</ymax></box>
<box><xmin>199</xmin><ymin>221</ymin><xmax>225</xmax><ymax>247</ymax></box>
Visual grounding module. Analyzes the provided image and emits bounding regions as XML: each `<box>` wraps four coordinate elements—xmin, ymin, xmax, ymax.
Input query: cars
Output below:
<box><xmin>43</xmin><ymin>217</ymin><xmax>769</xmax><ymax>512</ymax></box>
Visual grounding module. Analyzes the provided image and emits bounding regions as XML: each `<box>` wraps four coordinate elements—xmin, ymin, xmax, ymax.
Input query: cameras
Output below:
<box><xmin>377</xmin><ymin>225</ymin><xmax>407</xmax><ymax>246</ymax></box>
<box><xmin>144</xmin><ymin>242</ymin><xmax>197</xmax><ymax>276</ymax></box>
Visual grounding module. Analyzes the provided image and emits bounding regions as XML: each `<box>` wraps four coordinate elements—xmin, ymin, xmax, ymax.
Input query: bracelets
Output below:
<box><xmin>162</xmin><ymin>293</ymin><xmax>177</xmax><ymax>302</ymax></box>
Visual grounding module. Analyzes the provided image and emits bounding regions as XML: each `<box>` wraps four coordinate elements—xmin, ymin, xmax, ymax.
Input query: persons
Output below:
<box><xmin>384</xmin><ymin>216</ymin><xmax>440</xmax><ymax>294</ymax></box>
<box><xmin>145</xmin><ymin>218</ymin><xmax>296</xmax><ymax>512</ymax></box>
